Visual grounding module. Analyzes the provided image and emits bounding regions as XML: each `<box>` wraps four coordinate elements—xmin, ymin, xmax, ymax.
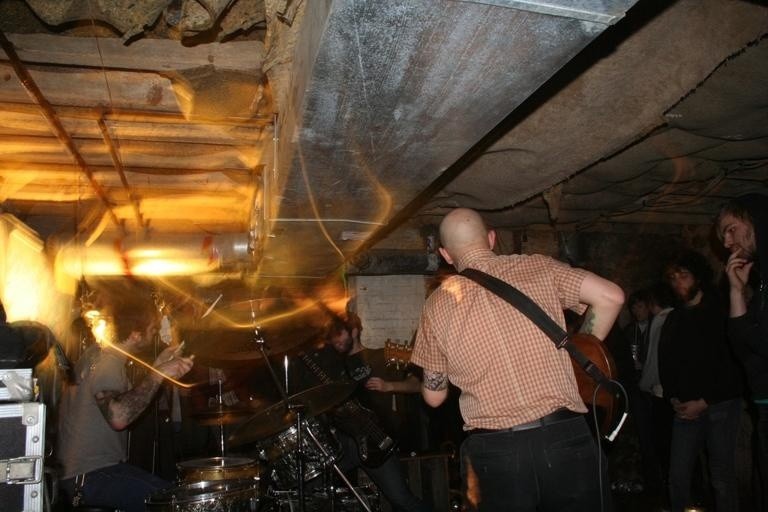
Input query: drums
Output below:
<box><xmin>144</xmin><ymin>476</ymin><xmax>260</xmax><ymax>512</ymax></box>
<box><xmin>255</xmin><ymin>414</ymin><xmax>344</xmax><ymax>490</ymax></box>
<box><xmin>176</xmin><ymin>457</ymin><xmax>262</xmax><ymax>511</ymax></box>
<box><xmin>259</xmin><ymin>482</ymin><xmax>381</xmax><ymax>512</ymax></box>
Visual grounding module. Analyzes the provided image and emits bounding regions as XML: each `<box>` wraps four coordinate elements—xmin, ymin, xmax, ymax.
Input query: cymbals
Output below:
<box><xmin>181</xmin><ymin>296</ymin><xmax>326</xmax><ymax>361</ymax></box>
<box><xmin>226</xmin><ymin>378</ymin><xmax>359</xmax><ymax>447</ymax></box>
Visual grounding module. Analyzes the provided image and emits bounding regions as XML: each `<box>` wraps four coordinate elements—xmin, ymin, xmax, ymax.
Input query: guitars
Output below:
<box><xmin>384</xmin><ymin>333</ymin><xmax>619</xmax><ymax>443</ymax></box>
<box><xmin>292</xmin><ymin>345</ymin><xmax>407</xmax><ymax>468</ymax></box>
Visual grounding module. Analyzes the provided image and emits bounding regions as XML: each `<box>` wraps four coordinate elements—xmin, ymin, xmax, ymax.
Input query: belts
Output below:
<box><xmin>459</xmin><ymin>405</ymin><xmax>588</xmax><ymax>435</ymax></box>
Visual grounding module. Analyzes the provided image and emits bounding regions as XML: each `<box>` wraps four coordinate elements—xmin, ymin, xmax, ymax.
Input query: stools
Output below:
<box><xmin>398</xmin><ymin>452</ymin><xmax>452</xmax><ymax>511</ymax></box>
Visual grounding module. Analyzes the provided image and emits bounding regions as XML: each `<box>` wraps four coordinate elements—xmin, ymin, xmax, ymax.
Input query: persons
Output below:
<box><xmin>658</xmin><ymin>248</ymin><xmax>746</xmax><ymax>511</ymax></box>
<box><xmin>297</xmin><ymin>310</ymin><xmax>435</xmax><ymax>512</ymax></box>
<box><xmin>714</xmin><ymin>191</ymin><xmax>767</xmax><ymax>511</ymax></box>
<box><xmin>620</xmin><ymin>290</ymin><xmax>661</xmax><ymax>499</ymax></box>
<box><xmin>410</xmin><ymin>204</ymin><xmax>627</xmax><ymax>512</ymax></box>
<box><xmin>54</xmin><ymin>296</ymin><xmax>197</xmax><ymax>510</ymax></box>
<box><xmin>637</xmin><ymin>283</ymin><xmax>675</xmax><ymax>485</ymax></box>
